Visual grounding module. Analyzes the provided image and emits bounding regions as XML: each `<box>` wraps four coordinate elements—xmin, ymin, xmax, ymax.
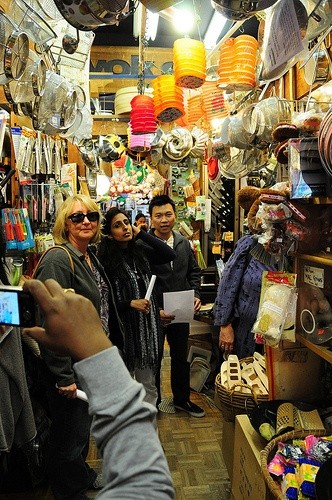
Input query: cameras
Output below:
<box><xmin>0</xmin><ymin>285</ymin><xmax>36</xmax><ymax>328</ymax></box>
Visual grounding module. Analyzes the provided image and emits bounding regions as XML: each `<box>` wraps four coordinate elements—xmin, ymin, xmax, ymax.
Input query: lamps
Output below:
<box><xmin>133</xmin><ymin>0</ymin><xmax>159</xmax><ymax>46</ymax></box>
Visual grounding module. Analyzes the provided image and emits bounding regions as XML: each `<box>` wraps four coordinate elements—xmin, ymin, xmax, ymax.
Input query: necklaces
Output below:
<box><xmin>86</xmin><ymin>254</ymin><xmax>89</xmax><ymax>259</ymax></box>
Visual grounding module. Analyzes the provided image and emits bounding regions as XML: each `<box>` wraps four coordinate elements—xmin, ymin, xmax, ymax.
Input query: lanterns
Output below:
<box><xmin>129</xmin><ymin>94</ymin><xmax>158</xmax><ymax>135</ymax></box>
<box><xmin>217</xmin><ymin>42</ymin><xmax>233</xmax><ymax>90</ymax></box>
<box><xmin>200</xmin><ymin>80</ymin><xmax>228</xmax><ymax>119</ymax></box>
<box><xmin>128</xmin><ymin>122</ymin><xmax>151</xmax><ymax>152</ymax></box>
<box><xmin>173</xmin><ymin>39</ymin><xmax>206</xmax><ymax>89</ymax></box>
<box><xmin>187</xmin><ymin>94</ymin><xmax>210</xmax><ymax>128</ymax></box>
<box><xmin>151</xmin><ymin>75</ymin><xmax>186</xmax><ymax>123</ymax></box>
<box><xmin>228</xmin><ymin>35</ymin><xmax>258</xmax><ymax>91</ymax></box>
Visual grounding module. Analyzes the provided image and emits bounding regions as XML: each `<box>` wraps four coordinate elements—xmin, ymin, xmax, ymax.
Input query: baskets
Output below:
<box><xmin>259</xmin><ymin>427</ymin><xmax>332</xmax><ymax>500</ymax></box>
<box><xmin>214</xmin><ymin>358</ymin><xmax>269</xmax><ymax>421</ymax></box>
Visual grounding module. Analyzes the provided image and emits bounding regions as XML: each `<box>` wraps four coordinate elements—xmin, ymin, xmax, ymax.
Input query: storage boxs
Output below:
<box><xmin>296</xmin><ymin>253</ymin><xmax>332</xmax><ymax>364</ymax></box>
<box><xmin>2</xmin><ymin>208</ymin><xmax>34</xmax><ymax>250</ymax></box>
<box><xmin>230</xmin><ymin>415</ymin><xmax>266</xmax><ymax>500</ymax></box>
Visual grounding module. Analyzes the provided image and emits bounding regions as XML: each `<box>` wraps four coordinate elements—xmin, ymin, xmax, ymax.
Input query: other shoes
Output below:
<box><xmin>174</xmin><ymin>398</ymin><xmax>205</xmax><ymax>418</ymax></box>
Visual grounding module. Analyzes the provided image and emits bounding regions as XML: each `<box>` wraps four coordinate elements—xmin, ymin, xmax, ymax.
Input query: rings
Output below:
<box><xmin>64</xmin><ymin>288</ymin><xmax>75</xmax><ymax>293</ymax></box>
<box><xmin>222</xmin><ymin>345</ymin><xmax>224</xmax><ymax>346</ymax></box>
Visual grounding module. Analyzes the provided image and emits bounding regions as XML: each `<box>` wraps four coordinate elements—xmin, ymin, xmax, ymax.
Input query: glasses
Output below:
<box><xmin>67</xmin><ymin>211</ymin><xmax>99</xmax><ymax>224</ymax></box>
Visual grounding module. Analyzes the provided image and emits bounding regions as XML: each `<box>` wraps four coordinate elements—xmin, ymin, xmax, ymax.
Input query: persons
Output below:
<box><xmin>32</xmin><ymin>194</ymin><xmax>126</xmax><ymax>500</ymax></box>
<box><xmin>93</xmin><ymin>207</ymin><xmax>177</xmax><ymax>454</ymax></box>
<box><xmin>143</xmin><ymin>195</ymin><xmax>206</xmax><ymax>417</ymax></box>
<box><xmin>214</xmin><ymin>233</ymin><xmax>277</xmax><ymax>494</ymax></box>
<box><xmin>0</xmin><ymin>279</ymin><xmax>175</xmax><ymax>500</ymax></box>
<box><xmin>133</xmin><ymin>213</ymin><xmax>148</xmax><ymax>233</ymax></box>
